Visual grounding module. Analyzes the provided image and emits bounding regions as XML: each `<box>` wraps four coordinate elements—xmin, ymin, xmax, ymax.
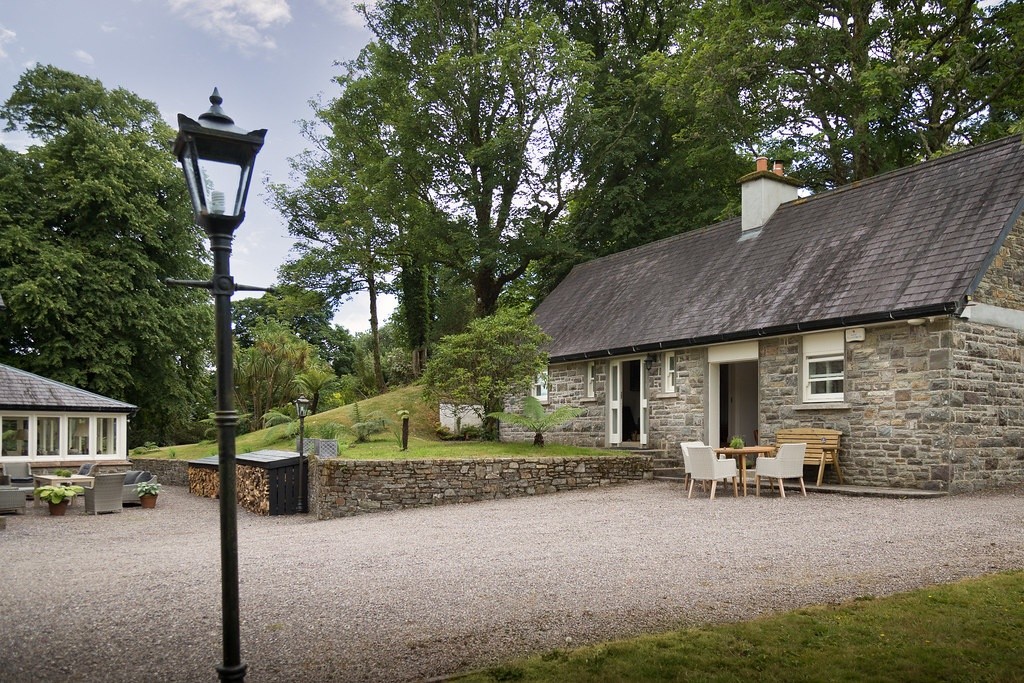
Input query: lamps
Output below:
<box><xmin>13</xmin><ymin>429</ymin><xmax>25</xmax><ymax>440</ymax></box>
<box><xmin>73</xmin><ymin>423</ymin><xmax>89</xmax><ymax>454</ymax></box>
<box><xmin>644</xmin><ymin>353</ymin><xmax>657</xmax><ymax>370</ymax></box>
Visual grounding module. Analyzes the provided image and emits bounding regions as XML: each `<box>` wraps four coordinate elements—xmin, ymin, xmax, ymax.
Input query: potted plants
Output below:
<box><xmin>729</xmin><ymin>437</ymin><xmax>745</xmax><ymax>449</ymax></box>
<box><xmin>132</xmin><ymin>482</ymin><xmax>162</xmax><ymax>507</ymax></box>
<box><xmin>33</xmin><ymin>485</ymin><xmax>84</xmax><ymax>515</ymax></box>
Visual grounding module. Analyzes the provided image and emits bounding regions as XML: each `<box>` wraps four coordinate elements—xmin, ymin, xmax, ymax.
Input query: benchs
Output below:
<box><xmin>760</xmin><ymin>429</ymin><xmax>843</xmax><ymax>486</ymax></box>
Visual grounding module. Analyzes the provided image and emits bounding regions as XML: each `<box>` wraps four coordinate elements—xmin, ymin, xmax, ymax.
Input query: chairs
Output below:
<box><xmin>0</xmin><ymin>473</ymin><xmax>26</xmax><ymax>516</ymax></box>
<box><xmin>85</xmin><ymin>473</ymin><xmax>126</xmax><ymax>515</ymax></box>
<box><xmin>77</xmin><ymin>463</ymin><xmax>99</xmax><ymax>476</ymax></box>
<box><xmin>756</xmin><ymin>443</ymin><xmax>808</xmax><ymax>498</ymax></box>
<box><xmin>2</xmin><ymin>463</ymin><xmax>34</xmax><ymax>500</ymax></box>
<box><xmin>680</xmin><ymin>440</ymin><xmax>727</xmax><ymax>491</ymax></box>
<box><xmin>687</xmin><ymin>445</ymin><xmax>739</xmax><ymax>500</ymax></box>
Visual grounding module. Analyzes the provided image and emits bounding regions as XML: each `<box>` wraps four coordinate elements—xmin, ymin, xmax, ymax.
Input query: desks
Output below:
<box><xmin>33</xmin><ymin>474</ymin><xmax>95</xmax><ymax>508</ymax></box>
<box><xmin>708</xmin><ymin>447</ymin><xmax>775</xmax><ymax>496</ymax></box>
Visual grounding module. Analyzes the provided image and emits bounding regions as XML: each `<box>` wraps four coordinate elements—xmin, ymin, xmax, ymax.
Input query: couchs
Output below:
<box><xmin>123</xmin><ymin>471</ymin><xmax>158</xmax><ymax>505</ymax></box>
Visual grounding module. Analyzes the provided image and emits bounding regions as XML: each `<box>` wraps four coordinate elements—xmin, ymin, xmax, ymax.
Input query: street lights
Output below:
<box><xmin>292</xmin><ymin>392</ymin><xmax>309</xmax><ymax>513</ymax></box>
<box><xmin>164</xmin><ymin>86</ymin><xmax>274</xmax><ymax>683</ymax></box>
